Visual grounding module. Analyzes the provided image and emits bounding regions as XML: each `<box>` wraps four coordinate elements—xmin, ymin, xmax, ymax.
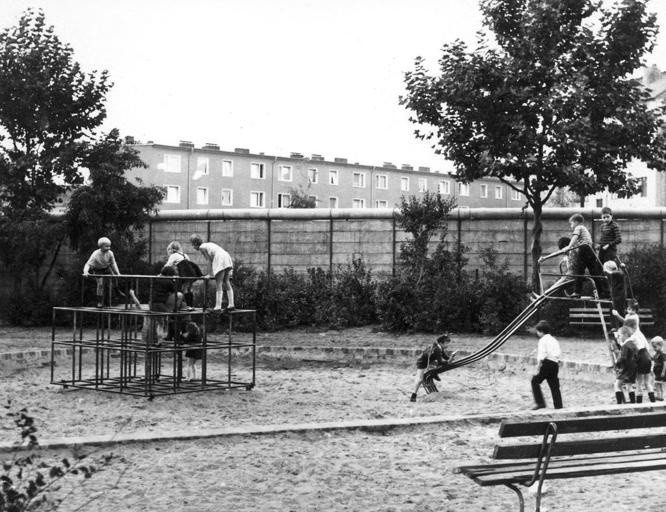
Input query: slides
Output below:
<box><xmin>422</xmin><ymin>280</ymin><xmax>574</xmax><ymax>394</ymax></box>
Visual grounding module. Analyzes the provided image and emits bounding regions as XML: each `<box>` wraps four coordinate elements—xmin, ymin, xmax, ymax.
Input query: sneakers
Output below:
<box><xmin>410</xmin><ymin>393</ymin><xmax>417</xmax><ymax>402</ymax></box>
<box><xmin>433</xmin><ymin>375</ymin><xmax>441</xmax><ymax>381</ymax></box>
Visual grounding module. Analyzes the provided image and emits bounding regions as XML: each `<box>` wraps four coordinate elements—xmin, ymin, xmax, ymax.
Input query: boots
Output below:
<box><xmin>616</xmin><ymin>391</ymin><xmax>654</xmax><ymax>403</ymax></box>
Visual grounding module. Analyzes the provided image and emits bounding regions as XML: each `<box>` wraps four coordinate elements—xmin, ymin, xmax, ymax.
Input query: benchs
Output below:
<box><xmin>568</xmin><ymin>307</ymin><xmax>655</xmax><ymax>331</ymax></box>
<box><xmin>452</xmin><ymin>413</ymin><xmax>666</xmax><ymax>511</ymax></box>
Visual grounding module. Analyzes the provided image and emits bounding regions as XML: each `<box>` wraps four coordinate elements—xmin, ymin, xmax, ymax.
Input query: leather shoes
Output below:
<box><xmin>210</xmin><ymin>307</ymin><xmax>235</xmax><ymax>313</ymax></box>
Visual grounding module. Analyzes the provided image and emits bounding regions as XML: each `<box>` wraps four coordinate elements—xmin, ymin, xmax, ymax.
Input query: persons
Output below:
<box><xmin>83</xmin><ymin>234</ymin><xmax>235</xmax><ymax>382</ymax></box>
<box><xmin>530</xmin><ymin>320</ymin><xmax>562</xmax><ymax>410</ymax></box>
<box><xmin>410</xmin><ymin>334</ymin><xmax>456</xmax><ymax>402</ymax></box>
<box><xmin>526</xmin><ymin>207</ymin><xmax>626</xmax><ymax>331</ymax></box>
<box><xmin>612</xmin><ymin>299</ymin><xmax>666</xmax><ymax>404</ymax></box>
